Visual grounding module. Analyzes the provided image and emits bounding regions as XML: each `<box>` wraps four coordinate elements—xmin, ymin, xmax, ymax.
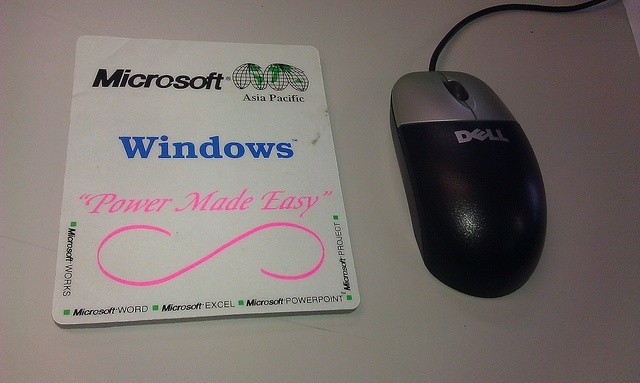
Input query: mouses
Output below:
<box><xmin>390</xmin><ymin>70</ymin><xmax>547</xmax><ymax>298</ymax></box>
